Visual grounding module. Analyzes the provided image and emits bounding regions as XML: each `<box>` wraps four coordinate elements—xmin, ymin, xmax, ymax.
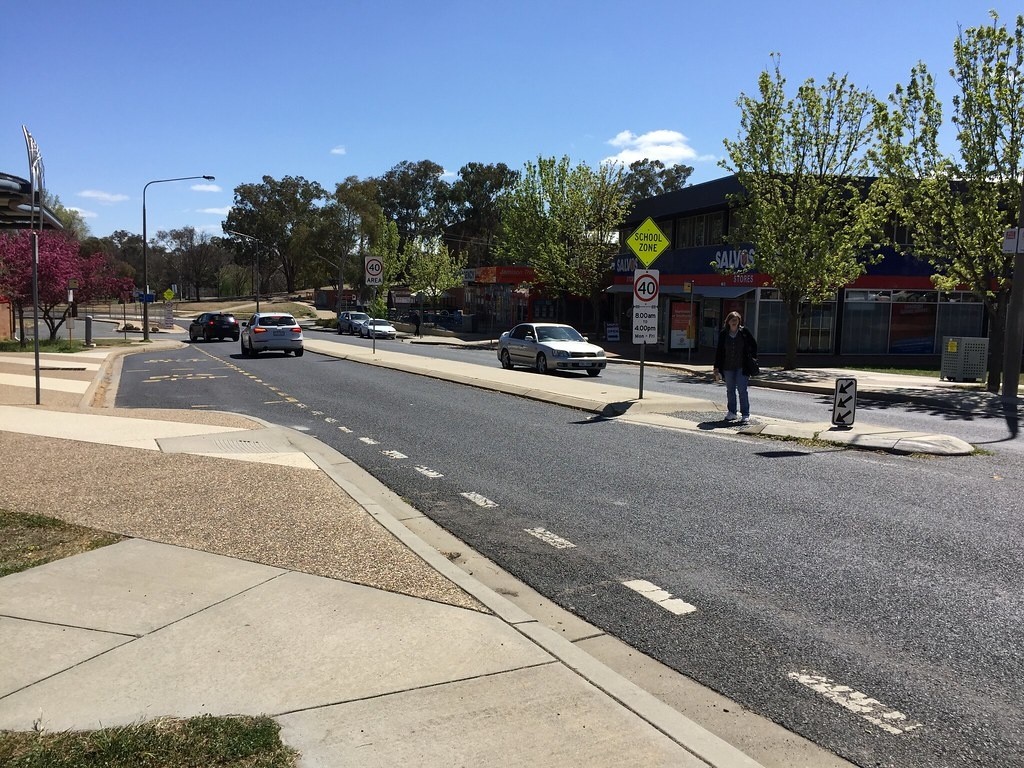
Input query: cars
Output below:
<box><xmin>360</xmin><ymin>318</ymin><xmax>396</xmax><ymax>339</ymax></box>
<box><xmin>497</xmin><ymin>323</ymin><xmax>607</xmax><ymax>377</ymax></box>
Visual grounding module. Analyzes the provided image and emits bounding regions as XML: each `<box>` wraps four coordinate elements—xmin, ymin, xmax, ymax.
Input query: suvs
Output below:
<box><xmin>189</xmin><ymin>312</ymin><xmax>241</xmax><ymax>341</ymax></box>
<box><xmin>241</xmin><ymin>312</ymin><xmax>304</xmax><ymax>356</ymax></box>
<box><xmin>336</xmin><ymin>311</ymin><xmax>372</xmax><ymax>336</ymax></box>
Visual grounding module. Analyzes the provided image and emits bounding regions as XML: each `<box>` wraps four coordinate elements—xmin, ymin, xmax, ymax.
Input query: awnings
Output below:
<box><xmin>604</xmin><ymin>284</ymin><xmax>756</xmax><ymax>298</ymax></box>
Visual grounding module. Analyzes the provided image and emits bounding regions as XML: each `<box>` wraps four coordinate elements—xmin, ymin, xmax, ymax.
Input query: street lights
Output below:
<box><xmin>140</xmin><ymin>175</ymin><xmax>216</xmax><ymax>343</ymax></box>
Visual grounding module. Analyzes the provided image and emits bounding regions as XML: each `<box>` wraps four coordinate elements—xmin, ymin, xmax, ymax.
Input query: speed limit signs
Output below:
<box><xmin>366</xmin><ymin>259</ymin><xmax>383</xmax><ymax>277</ymax></box>
<box><xmin>635</xmin><ymin>273</ymin><xmax>659</xmax><ymax>302</ymax></box>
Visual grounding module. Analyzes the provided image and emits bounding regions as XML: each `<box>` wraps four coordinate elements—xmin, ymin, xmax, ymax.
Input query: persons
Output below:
<box><xmin>713</xmin><ymin>311</ymin><xmax>758</xmax><ymax>422</ymax></box>
<box><xmin>414</xmin><ymin>310</ymin><xmax>420</xmax><ymax>336</ymax></box>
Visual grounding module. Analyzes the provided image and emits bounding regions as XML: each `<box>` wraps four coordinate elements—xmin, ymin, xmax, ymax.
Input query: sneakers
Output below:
<box><xmin>724</xmin><ymin>411</ymin><xmax>738</xmax><ymax>420</ymax></box>
<box><xmin>741</xmin><ymin>415</ymin><xmax>750</xmax><ymax>422</ymax></box>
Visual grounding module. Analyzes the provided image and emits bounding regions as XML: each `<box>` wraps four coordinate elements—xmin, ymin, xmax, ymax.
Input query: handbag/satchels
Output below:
<box><xmin>747</xmin><ymin>357</ymin><xmax>759</xmax><ymax>376</ymax></box>
